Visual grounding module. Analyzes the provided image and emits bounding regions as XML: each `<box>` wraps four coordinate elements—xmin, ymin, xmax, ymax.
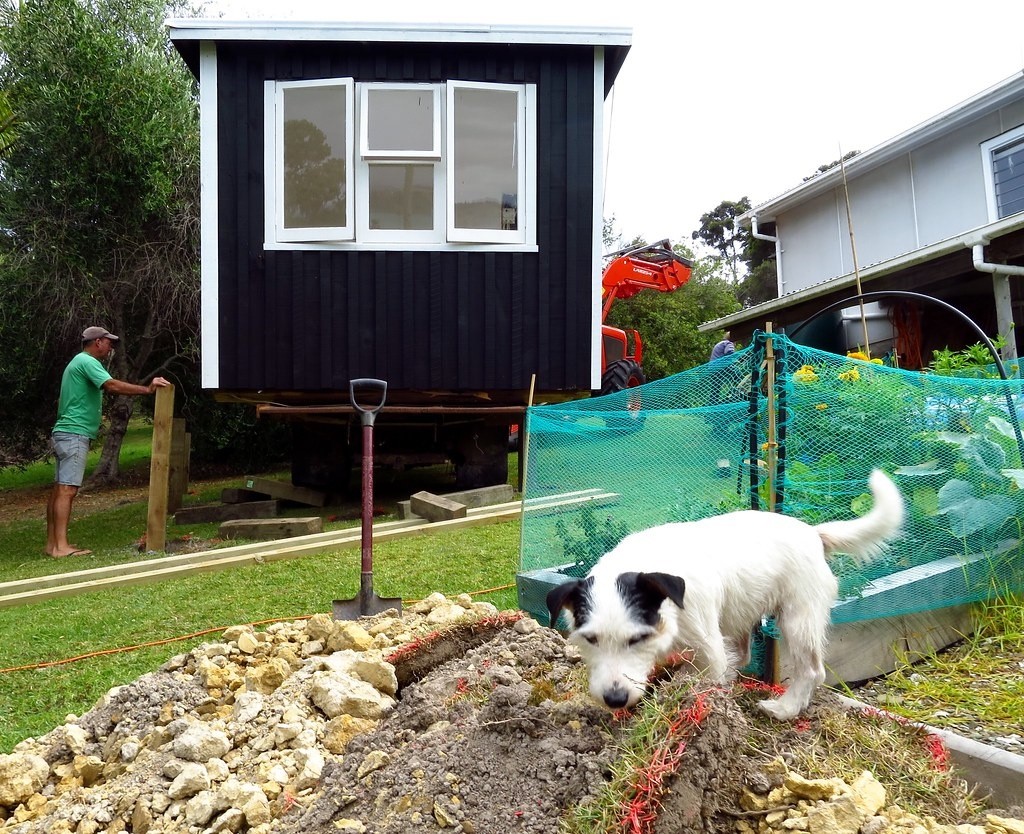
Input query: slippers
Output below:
<box><xmin>45</xmin><ymin>543</ymin><xmax>93</xmax><ymax>558</ymax></box>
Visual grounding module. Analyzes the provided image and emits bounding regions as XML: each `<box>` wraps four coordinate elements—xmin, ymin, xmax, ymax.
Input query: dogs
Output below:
<box><xmin>546</xmin><ymin>465</ymin><xmax>908</xmax><ymax>723</ymax></box>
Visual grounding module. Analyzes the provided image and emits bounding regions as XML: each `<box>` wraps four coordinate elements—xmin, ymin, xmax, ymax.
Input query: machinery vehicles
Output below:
<box><xmin>286</xmin><ymin>239</ymin><xmax>698</xmax><ymax>490</ymax></box>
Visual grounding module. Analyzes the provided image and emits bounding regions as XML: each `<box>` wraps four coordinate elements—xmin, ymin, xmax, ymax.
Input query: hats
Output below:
<box><xmin>81</xmin><ymin>326</ymin><xmax>119</xmax><ymax>341</ymax></box>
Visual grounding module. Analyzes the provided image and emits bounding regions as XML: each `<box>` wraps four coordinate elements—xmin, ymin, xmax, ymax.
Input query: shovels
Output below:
<box><xmin>331</xmin><ymin>377</ymin><xmax>402</xmax><ymax>619</ymax></box>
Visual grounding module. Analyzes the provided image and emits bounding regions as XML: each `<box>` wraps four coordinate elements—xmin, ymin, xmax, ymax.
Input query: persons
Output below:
<box><xmin>709</xmin><ymin>332</ymin><xmax>741</xmax><ymax>406</ymax></box>
<box><xmin>46</xmin><ymin>327</ymin><xmax>170</xmax><ymax>557</ymax></box>
